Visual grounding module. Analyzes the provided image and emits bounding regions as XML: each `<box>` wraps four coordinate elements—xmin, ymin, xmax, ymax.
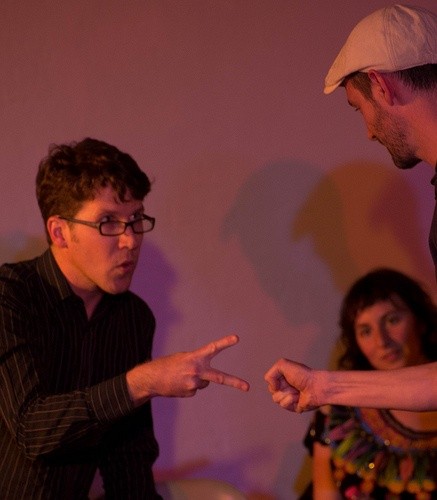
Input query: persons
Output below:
<box><xmin>0</xmin><ymin>134</ymin><xmax>251</xmax><ymax>499</ymax></box>
<box><xmin>262</xmin><ymin>5</ymin><xmax>436</xmax><ymax>416</ymax></box>
<box><xmin>293</xmin><ymin>264</ymin><xmax>437</xmax><ymax>499</ymax></box>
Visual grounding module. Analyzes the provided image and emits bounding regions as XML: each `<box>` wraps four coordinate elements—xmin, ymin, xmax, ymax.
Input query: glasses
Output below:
<box><xmin>56</xmin><ymin>213</ymin><xmax>155</xmax><ymax>236</ymax></box>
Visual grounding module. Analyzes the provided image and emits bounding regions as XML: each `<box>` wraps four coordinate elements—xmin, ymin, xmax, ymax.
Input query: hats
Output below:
<box><xmin>323</xmin><ymin>3</ymin><xmax>437</xmax><ymax>95</ymax></box>
